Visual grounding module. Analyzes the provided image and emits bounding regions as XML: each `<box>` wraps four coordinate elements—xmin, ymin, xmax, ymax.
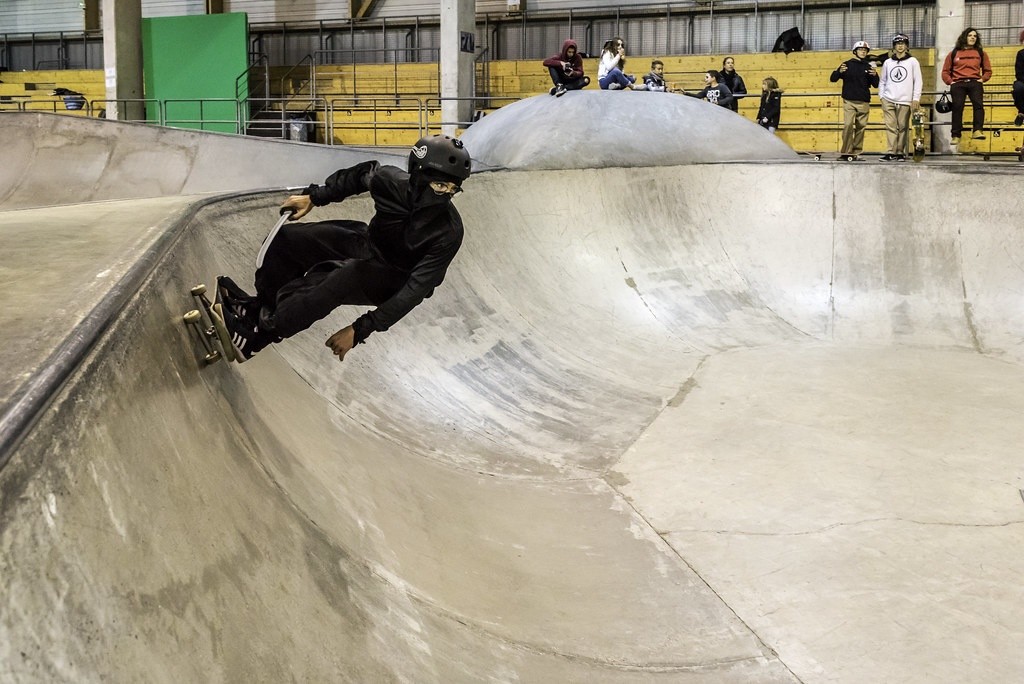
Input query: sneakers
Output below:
<box><xmin>220</xmin><ymin>299</ymin><xmax>272</xmax><ymax>363</ymax></box>
<box><xmin>218</xmin><ymin>276</ymin><xmax>249</xmax><ymax>307</ymax></box>
<box><xmin>556</xmin><ymin>84</ymin><xmax>567</xmax><ymax>96</ymax></box>
<box><xmin>879</xmin><ymin>154</ymin><xmax>896</xmax><ymax>161</ymax></box>
<box><xmin>972</xmin><ymin>130</ymin><xmax>986</xmax><ymax>139</ymax></box>
<box><xmin>950</xmin><ymin>137</ymin><xmax>960</xmax><ymax>144</ymax></box>
<box><xmin>897</xmin><ymin>155</ymin><xmax>904</xmax><ymax>161</ymax></box>
<box><xmin>549</xmin><ymin>87</ymin><xmax>556</xmax><ymax>95</ymax></box>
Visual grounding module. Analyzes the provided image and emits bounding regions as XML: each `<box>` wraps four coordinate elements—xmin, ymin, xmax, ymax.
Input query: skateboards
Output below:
<box><xmin>972</xmin><ymin>149</ymin><xmax>1024</xmax><ymax>161</ymax></box>
<box><xmin>804</xmin><ymin>151</ymin><xmax>866</xmax><ymax>161</ymax></box>
<box><xmin>910</xmin><ymin>107</ymin><xmax>926</xmax><ymax>162</ymax></box>
<box><xmin>183</xmin><ymin>275</ymin><xmax>237</xmax><ymax>367</ymax></box>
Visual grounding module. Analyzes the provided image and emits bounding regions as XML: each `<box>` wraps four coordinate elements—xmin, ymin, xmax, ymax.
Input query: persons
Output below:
<box><xmin>941</xmin><ymin>27</ymin><xmax>993</xmax><ymax>145</ymax></box>
<box><xmin>719</xmin><ymin>57</ymin><xmax>747</xmax><ymax>113</ymax></box>
<box><xmin>1011</xmin><ymin>30</ymin><xmax>1024</xmax><ymax>126</ymax></box>
<box><xmin>756</xmin><ymin>77</ymin><xmax>785</xmax><ymax>135</ymax></box>
<box><xmin>596</xmin><ymin>37</ymin><xmax>648</xmax><ymax>90</ymax></box>
<box><xmin>830</xmin><ymin>40</ymin><xmax>880</xmax><ymax>161</ymax></box>
<box><xmin>642</xmin><ymin>60</ymin><xmax>674</xmax><ymax>92</ymax></box>
<box><xmin>680</xmin><ymin>69</ymin><xmax>734</xmax><ymax>112</ymax></box>
<box><xmin>543</xmin><ymin>39</ymin><xmax>590</xmax><ymax>96</ymax></box>
<box><xmin>214</xmin><ymin>136</ymin><xmax>472</xmax><ymax>364</ymax></box>
<box><xmin>878</xmin><ymin>34</ymin><xmax>923</xmax><ymax>162</ymax></box>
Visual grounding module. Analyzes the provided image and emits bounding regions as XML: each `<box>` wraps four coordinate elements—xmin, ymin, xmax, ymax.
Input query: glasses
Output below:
<box><xmin>432</xmin><ymin>182</ymin><xmax>463</xmax><ymax>199</ymax></box>
<box><xmin>617</xmin><ymin>43</ymin><xmax>624</xmax><ymax>47</ymax></box>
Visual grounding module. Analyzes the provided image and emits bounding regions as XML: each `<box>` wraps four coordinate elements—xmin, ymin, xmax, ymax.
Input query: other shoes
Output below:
<box><xmin>633</xmin><ymin>84</ymin><xmax>647</xmax><ymax>91</ymax></box>
<box><xmin>608</xmin><ymin>83</ymin><xmax>621</xmax><ymax>89</ymax></box>
<box><xmin>837</xmin><ymin>155</ymin><xmax>852</xmax><ymax>161</ymax></box>
<box><xmin>1015</xmin><ymin>115</ymin><xmax>1024</xmax><ymax>125</ymax></box>
<box><xmin>853</xmin><ymin>157</ymin><xmax>865</xmax><ymax>161</ymax></box>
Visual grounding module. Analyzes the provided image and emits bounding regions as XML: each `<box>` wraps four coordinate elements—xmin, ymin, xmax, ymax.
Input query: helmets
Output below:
<box><xmin>852</xmin><ymin>41</ymin><xmax>870</xmax><ymax>54</ymax></box>
<box><xmin>893</xmin><ymin>34</ymin><xmax>909</xmax><ymax>44</ymax></box>
<box><xmin>408</xmin><ymin>133</ymin><xmax>471</xmax><ymax>181</ymax></box>
<box><xmin>936</xmin><ymin>101</ymin><xmax>953</xmax><ymax>113</ymax></box>
